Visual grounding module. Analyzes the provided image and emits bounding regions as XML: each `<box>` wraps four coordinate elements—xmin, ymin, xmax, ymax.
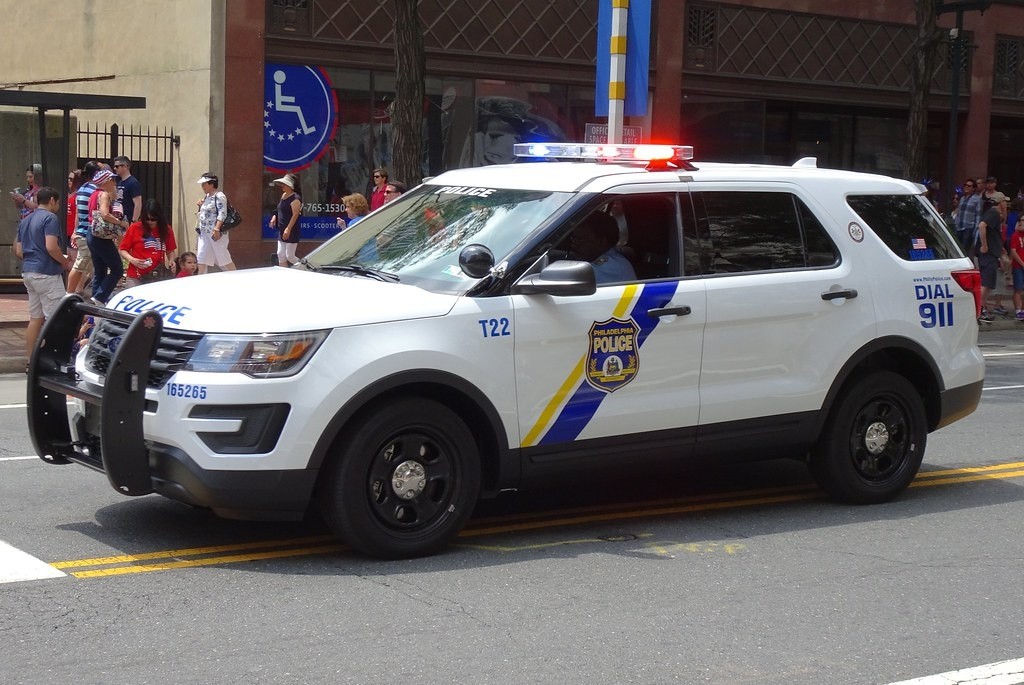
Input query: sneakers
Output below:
<box><xmin>994</xmin><ymin>305</ymin><xmax>1008</xmax><ymax>315</ymax></box>
<box><xmin>980</xmin><ymin>306</ymin><xmax>995</xmax><ymax>323</ymax></box>
<box><xmin>1014</xmin><ymin>310</ymin><xmax>1024</xmax><ymax>321</ymax></box>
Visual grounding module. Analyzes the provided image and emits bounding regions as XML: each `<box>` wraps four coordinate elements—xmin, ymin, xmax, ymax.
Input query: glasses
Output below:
<box><xmin>373</xmin><ymin>175</ymin><xmax>381</xmax><ymax>178</ymax></box>
<box><xmin>113</xmin><ymin>165</ymin><xmax>124</xmax><ymax>168</ymax></box>
<box><xmin>384</xmin><ymin>190</ymin><xmax>397</xmax><ymax>194</ymax></box>
<box><xmin>68</xmin><ymin>177</ymin><xmax>74</xmax><ymax>182</ymax></box>
<box><xmin>964</xmin><ymin>184</ymin><xmax>972</xmax><ymax>188</ymax></box>
<box><xmin>952</xmin><ymin>197</ymin><xmax>958</xmax><ymax>201</ymax></box>
<box><xmin>146</xmin><ymin>217</ymin><xmax>157</xmax><ymax>221</ymax></box>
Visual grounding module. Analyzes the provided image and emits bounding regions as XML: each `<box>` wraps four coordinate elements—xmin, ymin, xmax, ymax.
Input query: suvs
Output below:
<box><xmin>27</xmin><ymin>141</ymin><xmax>987</xmax><ymax>564</ymax></box>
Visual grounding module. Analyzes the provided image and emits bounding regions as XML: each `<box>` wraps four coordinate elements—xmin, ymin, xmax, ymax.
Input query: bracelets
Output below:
<box><xmin>131</xmin><ymin>219</ymin><xmax>135</xmax><ymax>224</ymax></box>
<box><xmin>23</xmin><ymin>198</ymin><xmax>27</xmax><ymax>203</ymax></box>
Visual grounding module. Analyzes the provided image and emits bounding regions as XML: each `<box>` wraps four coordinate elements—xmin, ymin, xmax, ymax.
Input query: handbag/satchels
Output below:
<box><xmin>215</xmin><ymin>191</ymin><xmax>242</xmax><ymax>231</ymax></box>
<box><xmin>92</xmin><ymin>191</ymin><xmax>120</xmax><ymax>239</ymax></box>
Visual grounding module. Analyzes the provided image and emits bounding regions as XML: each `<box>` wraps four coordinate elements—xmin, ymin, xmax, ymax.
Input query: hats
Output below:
<box><xmin>93</xmin><ymin>169</ymin><xmax>118</xmax><ymax>184</ymax></box>
<box><xmin>986</xmin><ymin>177</ymin><xmax>997</xmax><ymax>182</ymax></box>
<box><xmin>272</xmin><ymin>175</ymin><xmax>294</xmax><ymax>190</ymax></box>
<box><xmin>991</xmin><ymin>192</ymin><xmax>1010</xmax><ymax>203</ymax></box>
<box><xmin>197</xmin><ymin>177</ymin><xmax>216</xmax><ymax>183</ymax></box>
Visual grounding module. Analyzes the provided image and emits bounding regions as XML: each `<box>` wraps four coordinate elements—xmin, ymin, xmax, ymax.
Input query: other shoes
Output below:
<box><xmin>90</xmin><ymin>297</ymin><xmax>105</xmax><ymax>308</ymax></box>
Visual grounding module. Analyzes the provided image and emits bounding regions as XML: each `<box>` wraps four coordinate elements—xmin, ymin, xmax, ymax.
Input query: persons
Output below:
<box><xmin>567</xmin><ymin>209</ymin><xmax>637</xmax><ymax>280</ymax></box>
<box><xmin>369</xmin><ymin>170</ymin><xmax>390</xmax><ymax>212</ymax></box>
<box><xmin>194</xmin><ymin>171</ymin><xmax>243</xmax><ymax>275</ymax></box>
<box><xmin>919</xmin><ymin>175</ymin><xmax>1024</xmax><ymax>244</ymax></box>
<box><xmin>67</xmin><ymin>168</ymin><xmax>85</xmax><ymax>275</ymax></box>
<box><xmin>1010</xmin><ymin>213</ymin><xmax>1024</xmax><ymax>322</ymax></box>
<box><xmin>173</xmin><ymin>252</ymin><xmax>200</xmax><ymax>278</ymax></box>
<box><xmin>111</xmin><ymin>156</ymin><xmax>143</xmax><ymax>289</ymax></box>
<box><xmin>10</xmin><ymin>164</ymin><xmax>42</xmax><ymax>258</ymax></box>
<box><xmin>977</xmin><ymin>192</ymin><xmax>1012</xmax><ymax>323</ymax></box>
<box><xmin>119</xmin><ymin>198</ymin><xmax>178</xmax><ymax>289</ymax></box>
<box><xmin>16</xmin><ymin>183</ymin><xmax>70</xmax><ymax>376</ymax></box>
<box><xmin>336</xmin><ymin>193</ymin><xmax>380</xmax><ymax>265</ymax></box>
<box><xmin>993</xmin><ymin>243</ymin><xmax>1011</xmax><ymax>315</ymax></box>
<box><xmin>373</xmin><ymin>179</ymin><xmax>422</xmax><ymax>262</ymax></box>
<box><xmin>269</xmin><ymin>172</ymin><xmax>304</xmax><ymax>266</ymax></box>
<box><xmin>954</xmin><ymin>179</ymin><xmax>983</xmax><ymax>270</ymax></box>
<box><xmin>84</xmin><ymin>169</ymin><xmax>129</xmax><ymax>308</ymax></box>
<box><xmin>65</xmin><ymin>160</ymin><xmax>106</xmax><ymax>294</ymax></box>
